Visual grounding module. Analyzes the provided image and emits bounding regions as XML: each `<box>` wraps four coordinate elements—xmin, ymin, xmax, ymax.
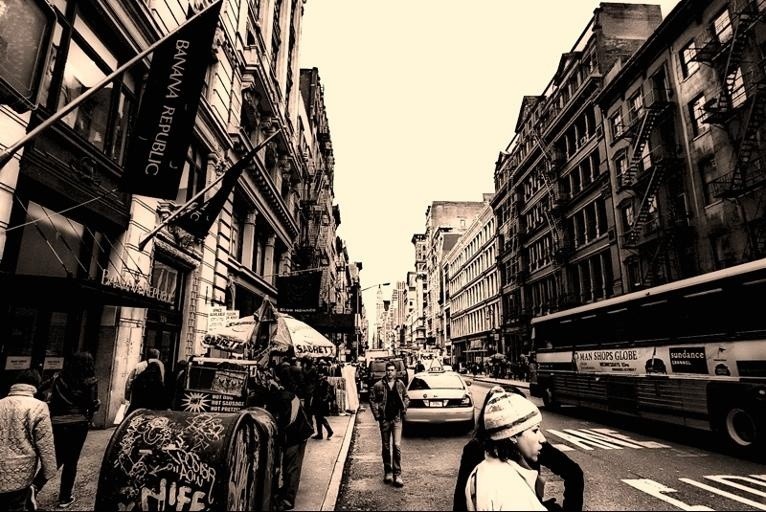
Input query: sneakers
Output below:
<box><xmin>274</xmin><ymin>500</ymin><xmax>294</xmax><ymax>511</ymax></box>
<box><xmin>59</xmin><ymin>495</ymin><xmax>75</xmax><ymax>508</ymax></box>
<box><xmin>26</xmin><ymin>485</ymin><xmax>38</xmax><ymax>511</ymax></box>
<box><xmin>384</xmin><ymin>471</ymin><xmax>403</xmax><ymax>487</ymax></box>
<box><xmin>312</xmin><ymin>431</ymin><xmax>333</xmax><ymax>439</ymax></box>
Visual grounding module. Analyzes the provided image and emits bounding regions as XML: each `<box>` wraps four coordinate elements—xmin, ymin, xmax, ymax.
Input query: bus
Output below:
<box><xmin>522</xmin><ymin>255</ymin><xmax>765</xmax><ymax>462</ymax></box>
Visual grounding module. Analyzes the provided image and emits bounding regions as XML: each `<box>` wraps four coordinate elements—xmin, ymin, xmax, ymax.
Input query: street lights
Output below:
<box><xmin>356</xmin><ymin>282</ymin><xmax>391</xmax><ymax>361</ymax></box>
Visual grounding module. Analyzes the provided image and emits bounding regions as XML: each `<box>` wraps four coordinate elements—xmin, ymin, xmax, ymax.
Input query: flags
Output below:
<box><xmin>111</xmin><ymin>1</ymin><xmax>224</xmax><ymax>203</ymax></box>
<box><xmin>165</xmin><ymin>144</ymin><xmax>265</xmax><ymax>245</ymax></box>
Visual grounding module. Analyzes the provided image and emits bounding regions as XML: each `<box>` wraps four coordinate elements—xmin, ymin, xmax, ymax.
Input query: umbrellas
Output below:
<box><xmin>201</xmin><ymin>308</ymin><xmax>339</xmax><ymax>362</ymax></box>
<box><xmin>247</xmin><ymin>292</ymin><xmax>279</xmax><ymax>367</ymax></box>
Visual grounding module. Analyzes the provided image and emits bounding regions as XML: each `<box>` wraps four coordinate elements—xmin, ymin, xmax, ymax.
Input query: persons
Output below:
<box><xmin>452</xmin><ymin>382</ymin><xmax>585</xmax><ymax>512</ymax></box>
<box><xmin>368</xmin><ymin>361</ymin><xmax>411</xmax><ymax>486</ymax></box>
<box><xmin>22</xmin><ymin>347</ymin><xmax>101</xmax><ymax>511</ymax></box>
<box><xmin>126</xmin><ymin>347</ymin><xmax>165</xmax><ymax>420</ymax></box>
<box><xmin>268</xmin><ymin>356</ymin><xmax>335</xmax><ymax>440</ymax></box>
<box><xmin>414</xmin><ymin>360</ymin><xmax>425</xmax><ymax>373</ymax></box>
<box><xmin>452</xmin><ymin>352</ymin><xmax>536</xmax><ymax>382</ymax></box>
<box><xmin>464</xmin><ymin>384</ymin><xmax>552</xmax><ymax>511</ymax></box>
<box><xmin>0</xmin><ymin>366</ymin><xmax>58</xmax><ymax>511</ymax></box>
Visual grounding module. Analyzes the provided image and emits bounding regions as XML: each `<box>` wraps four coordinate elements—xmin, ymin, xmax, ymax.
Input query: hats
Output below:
<box><xmin>484</xmin><ymin>386</ymin><xmax>543</xmax><ymax>440</ymax></box>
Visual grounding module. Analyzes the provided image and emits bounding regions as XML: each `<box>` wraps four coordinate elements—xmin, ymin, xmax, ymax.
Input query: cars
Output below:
<box><xmin>360</xmin><ymin>346</ymin><xmax>476</xmax><ymax>433</ymax></box>
